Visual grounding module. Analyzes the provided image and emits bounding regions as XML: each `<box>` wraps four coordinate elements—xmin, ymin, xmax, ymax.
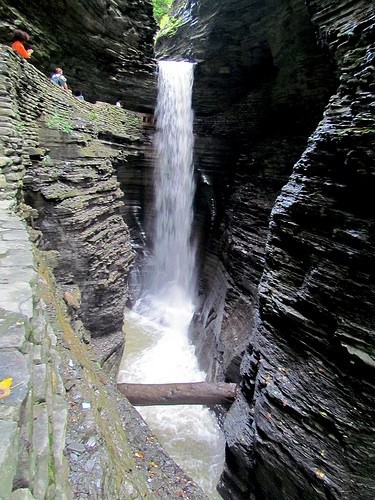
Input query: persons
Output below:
<box><xmin>11</xmin><ymin>28</ymin><xmax>34</xmax><ymax>59</ymax></box>
<box><xmin>51</xmin><ymin>68</ymin><xmax>69</xmax><ymax>91</ymax></box>
<box><xmin>116</xmin><ymin>98</ymin><xmax>123</xmax><ymax>107</ymax></box>
<box><xmin>73</xmin><ymin>89</ymin><xmax>84</xmax><ymax>100</ymax></box>
